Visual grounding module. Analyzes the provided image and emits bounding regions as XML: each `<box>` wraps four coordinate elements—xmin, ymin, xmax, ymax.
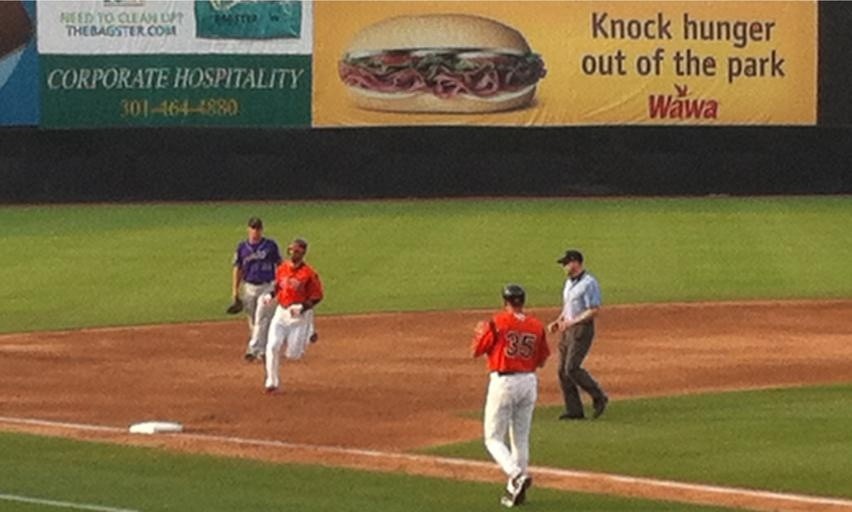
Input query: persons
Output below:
<box><xmin>547</xmin><ymin>249</ymin><xmax>609</xmax><ymax>420</ymax></box>
<box><xmin>258</xmin><ymin>238</ymin><xmax>325</xmax><ymax>393</ymax></box>
<box><xmin>227</xmin><ymin>215</ymin><xmax>281</xmax><ymax>362</ymax></box>
<box><xmin>471</xmin><ymin>283</ymin><xmax>550</xmax><ymax>508</ymax></box>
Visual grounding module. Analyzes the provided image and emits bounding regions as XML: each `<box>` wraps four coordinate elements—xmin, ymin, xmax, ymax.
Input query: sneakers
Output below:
<box><xmin>265</xmin><ymin>384</ymin><xmax>274</xmax><ymax>391</ymax></box>
<box><xmin>245</xmin><ymin>352</ymin><xmax>258</xmax><ymax>359</ymax></box>
<box><xmin>558</xmin><ymin>412</ymin><xmax>583</xmax><ymax>420</ymax></box>
<box><xmin>500</xmin><ymin>472</ymin><xmax>532</xmax><ymax>509</ymax></box>
<box><xmin>593</xmin><ymin>398</ymin><xmax>607</xmax><ymax>418</ymax></box>
<box><xmin>311</xmin><ymin>333</ymin><xmax>318</xmax><ymax>343</ymax></box>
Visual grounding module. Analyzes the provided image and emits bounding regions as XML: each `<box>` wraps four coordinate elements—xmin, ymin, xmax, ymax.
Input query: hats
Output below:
<box><xmin>558</xmin><ymin>251</ymin><xmax>583</xmax><ymax>263</ymax></box>
<box><xmin>249</xmin><ymin>218</ymin><xmax>263</xmax><ymax>225</ymax></box>
<box><xmin>289</xmin><ymin>240</ymin><xmax>307</xmax><ymax>252</ymax></box>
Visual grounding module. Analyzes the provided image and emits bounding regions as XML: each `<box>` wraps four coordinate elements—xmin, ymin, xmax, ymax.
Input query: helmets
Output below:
<box><xmin>503</xmin><ymin>286</ymin><xmax>523</xmax><ymax>299</ymax></box>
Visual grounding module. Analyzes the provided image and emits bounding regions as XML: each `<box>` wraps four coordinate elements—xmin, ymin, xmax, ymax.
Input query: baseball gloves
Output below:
<box><xmin>227</xmin><ymin>299</ymin><xmax>243</xmax><ymax>313</ymax></box>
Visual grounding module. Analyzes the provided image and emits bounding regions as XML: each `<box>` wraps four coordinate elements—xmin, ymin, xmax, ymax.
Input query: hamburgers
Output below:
<box><xmin>339</xmin><ymin>15</ymin><xmax>547</xmax><ymax>112</ymax></box>
